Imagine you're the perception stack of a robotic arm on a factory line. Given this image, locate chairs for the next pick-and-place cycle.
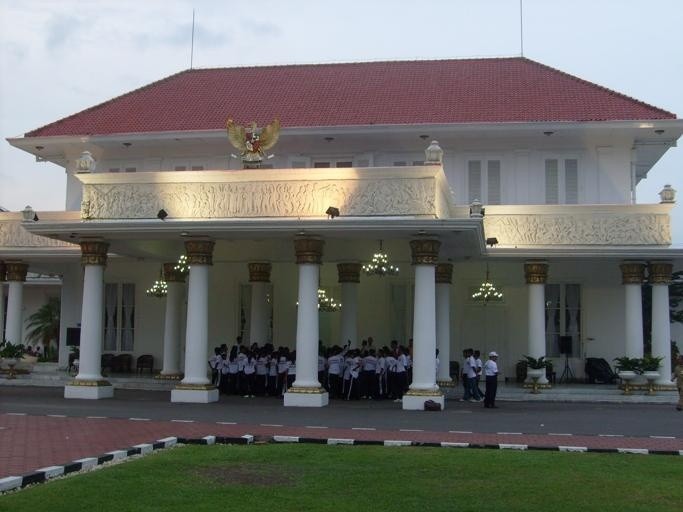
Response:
[67,352,153,375]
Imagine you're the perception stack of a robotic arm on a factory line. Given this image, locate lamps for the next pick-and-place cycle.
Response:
[469,259,504,307]
[156,209,168,221]
[142,261,168,299]
[484,237,498,248]
[325,206,339,220]
[294,264,343,313]
[359,239,400,278]
[173,244,189,277]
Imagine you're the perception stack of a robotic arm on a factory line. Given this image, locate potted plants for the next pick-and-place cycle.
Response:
[635,354,663,396]
[613,355,640,396]
[0,341,26,379]
[516,351,553,394]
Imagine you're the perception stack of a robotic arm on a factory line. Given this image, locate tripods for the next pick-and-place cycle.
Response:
[559,356,577,384]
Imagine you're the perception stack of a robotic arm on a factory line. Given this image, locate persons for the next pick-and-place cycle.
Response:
[25,345,34,354]
[670,355,683,410]
[208,336,413,404]
[33,346,43,361]
[459,348,485,403]
[483,351,499,408]
[436,349,439,384]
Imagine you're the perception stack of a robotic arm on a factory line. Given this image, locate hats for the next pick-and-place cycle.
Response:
[488,352,499,357]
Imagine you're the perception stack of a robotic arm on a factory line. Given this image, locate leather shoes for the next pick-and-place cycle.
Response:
[487,404,498,408]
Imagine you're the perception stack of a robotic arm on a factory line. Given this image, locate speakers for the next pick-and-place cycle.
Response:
[559,336,573,354]
[66,328,81,346]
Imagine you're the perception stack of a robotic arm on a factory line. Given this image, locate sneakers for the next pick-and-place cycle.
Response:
[329,392,403,404]
[460,394,485,402]
[217,389,283,400]
